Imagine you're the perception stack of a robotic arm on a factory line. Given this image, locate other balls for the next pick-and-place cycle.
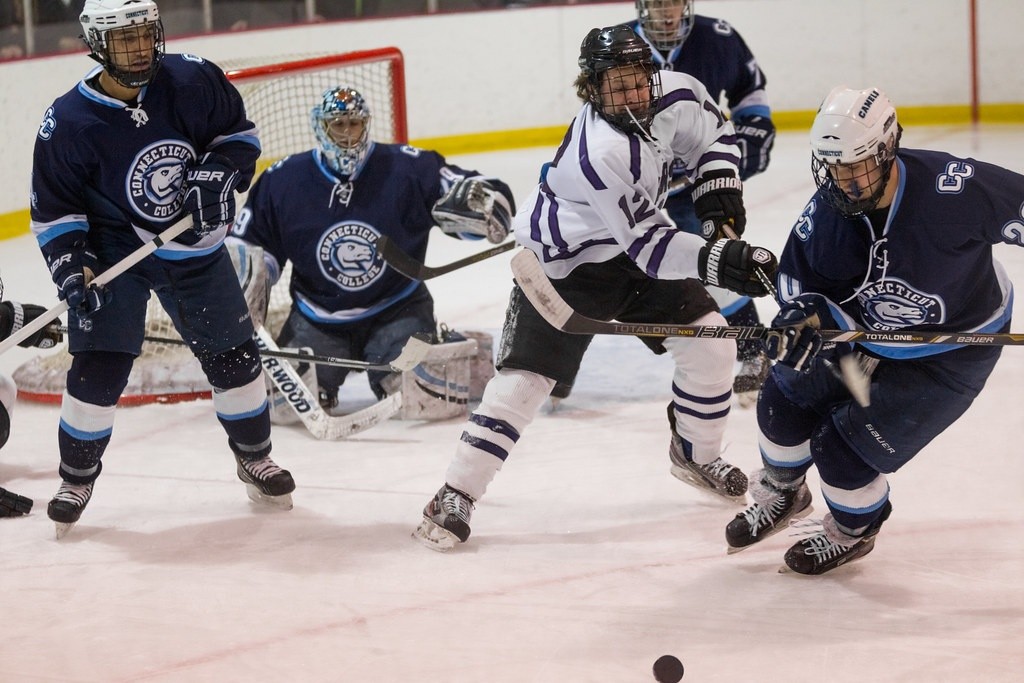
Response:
[652,654,685,683]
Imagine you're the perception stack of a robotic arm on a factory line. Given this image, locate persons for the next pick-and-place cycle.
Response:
[412,13,783,549]
[0,276,62,516]
[222,84,517,424]
[724,82,1023,576]
[26,0,296,539]
[620,0,776,408]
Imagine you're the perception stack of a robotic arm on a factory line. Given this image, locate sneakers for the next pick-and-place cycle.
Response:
[410,482,476,554]
[776,498,893,576]
[46,476,95,541]
[233,450,296,511]
[724,475,814,555]
[666,400,749,506]
[733,349,774,409]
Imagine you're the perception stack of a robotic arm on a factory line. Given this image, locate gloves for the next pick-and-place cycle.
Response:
[760,302,825,375]
[46,246,114,335]
[697,236,780,299]
[3,299,63,349]
[735,115,775,181]
[682,170,747,241]
[179,152,244,240]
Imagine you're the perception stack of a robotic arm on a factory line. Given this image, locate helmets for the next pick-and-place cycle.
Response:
[808,84,904,223]
[78,0,166,89]
[576,24,663,135]
[635,0,696,51]
[310,86,373,176]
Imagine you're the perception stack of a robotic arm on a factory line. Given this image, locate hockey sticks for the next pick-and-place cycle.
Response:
[374,180,691,285]
[46,321,437,375]
[250,320,404,445]
[1,211,196,358]
[510,244,1024,351]
[720,221,874,409]
